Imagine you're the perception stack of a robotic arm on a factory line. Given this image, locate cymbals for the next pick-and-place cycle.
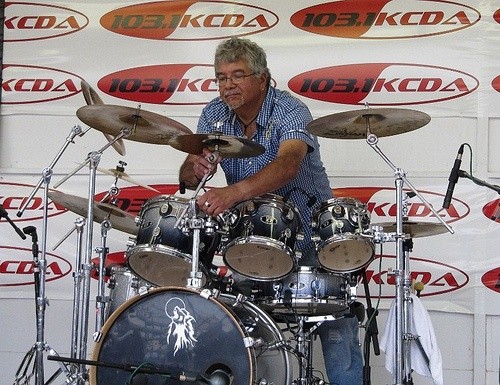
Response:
[80,80,127,156]
[75,161,162,193]
[167,133,267,158]
[48,193,141,235]
[305,109,431,139]
[368,222,452,238]
[76,105,193,145]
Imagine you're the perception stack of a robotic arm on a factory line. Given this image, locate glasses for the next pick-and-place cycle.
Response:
[213,72,255,86]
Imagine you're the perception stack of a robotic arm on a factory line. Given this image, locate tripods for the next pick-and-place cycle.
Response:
[13,125,90,385]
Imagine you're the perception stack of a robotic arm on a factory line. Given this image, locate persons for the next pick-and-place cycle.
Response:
[179,38,365,385]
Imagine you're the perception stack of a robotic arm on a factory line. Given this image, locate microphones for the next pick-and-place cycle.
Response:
[207,368,230,385]
[442,145,464,208]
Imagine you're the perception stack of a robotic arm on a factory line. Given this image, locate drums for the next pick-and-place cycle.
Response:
[221,191,302,282]
[91,285,294,385]
[103,267,151,324]
[310,196,375,273]
[123,194,222,289]
[251,265,354,323]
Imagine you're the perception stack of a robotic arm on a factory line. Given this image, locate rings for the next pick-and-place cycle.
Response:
[205,201,210,207]
[197,156,201,160]
